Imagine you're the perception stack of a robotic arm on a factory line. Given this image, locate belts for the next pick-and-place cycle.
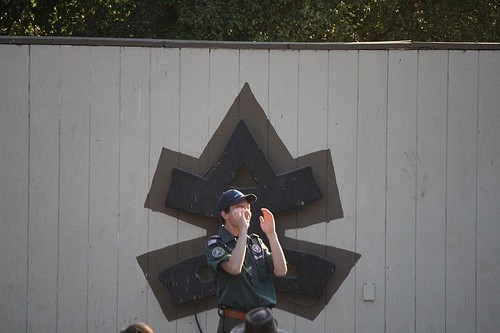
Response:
[224,309,246,320]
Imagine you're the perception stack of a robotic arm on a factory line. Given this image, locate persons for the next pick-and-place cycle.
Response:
[204,188,288,333]
[119,320,154,333]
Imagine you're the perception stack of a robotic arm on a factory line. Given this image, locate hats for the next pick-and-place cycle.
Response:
[230,307,291,333]
[218,189,257,211]
